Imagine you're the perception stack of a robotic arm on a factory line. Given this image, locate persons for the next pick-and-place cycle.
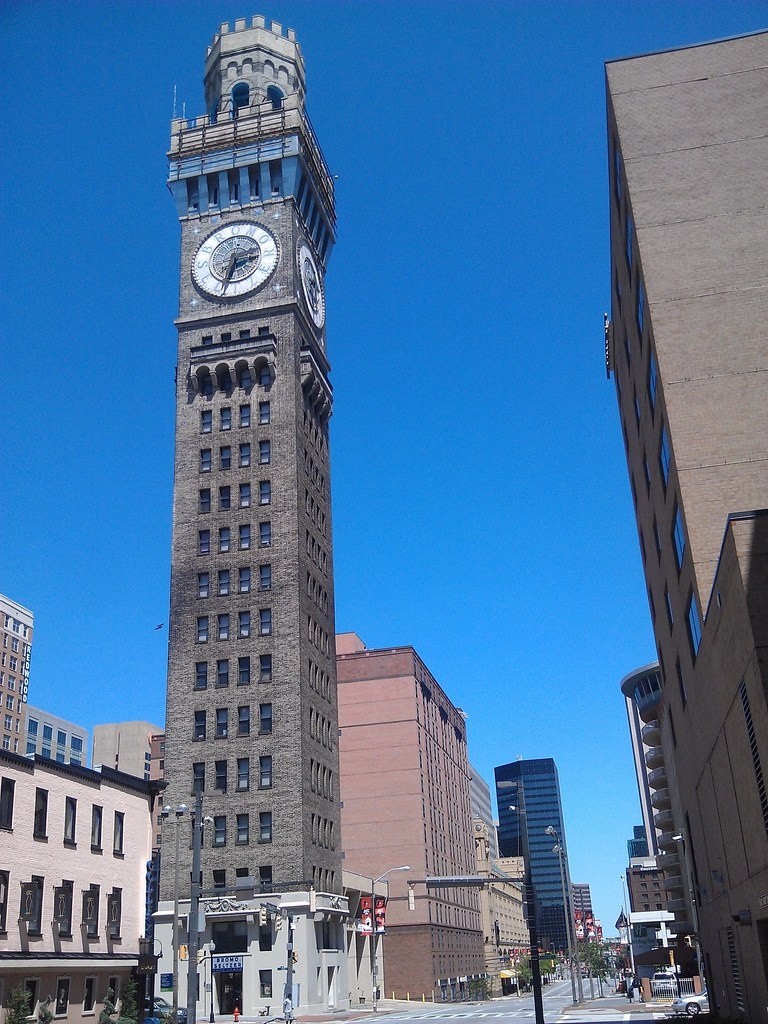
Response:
[283,993,294,1024]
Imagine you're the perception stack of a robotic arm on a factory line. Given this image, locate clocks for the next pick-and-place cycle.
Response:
[190,220,280,301]
[295,235,326,331]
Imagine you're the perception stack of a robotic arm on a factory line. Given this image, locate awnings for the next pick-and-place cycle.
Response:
[500,969,522,978]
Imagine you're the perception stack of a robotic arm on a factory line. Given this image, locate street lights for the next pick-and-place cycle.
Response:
[162,803,189,1023]
[208,939,217,1022]
[544,825,585,1007]
[371,866,410,1012]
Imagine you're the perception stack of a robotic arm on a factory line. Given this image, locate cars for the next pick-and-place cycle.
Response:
[143,994,187,1024]
[651,973,677,996]
[670,988,710,1015]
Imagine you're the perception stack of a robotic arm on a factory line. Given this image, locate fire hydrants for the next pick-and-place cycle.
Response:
[233,1006,241,1022]
